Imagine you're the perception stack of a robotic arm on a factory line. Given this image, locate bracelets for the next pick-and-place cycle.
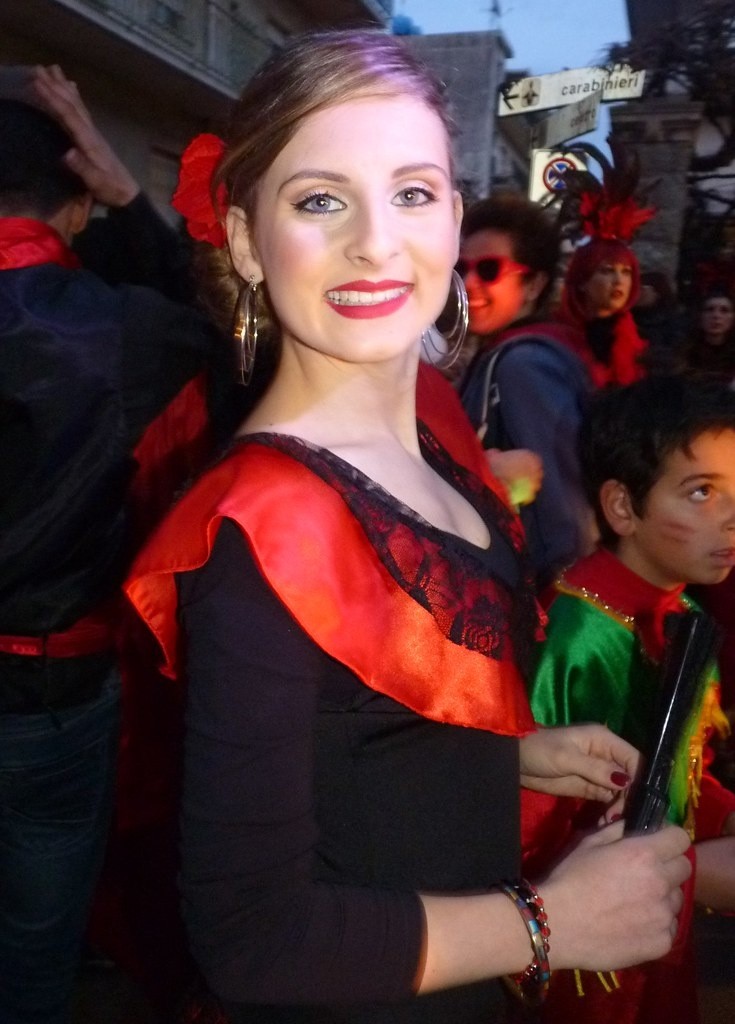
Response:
[489,882,551,1007]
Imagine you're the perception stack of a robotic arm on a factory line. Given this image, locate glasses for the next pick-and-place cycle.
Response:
[455,255,536,286]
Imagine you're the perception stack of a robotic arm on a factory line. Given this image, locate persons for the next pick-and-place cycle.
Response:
[530,372,735,1024]
[121,31,691,1024]
[1,65,224,1024]
[440,197,734,583]
